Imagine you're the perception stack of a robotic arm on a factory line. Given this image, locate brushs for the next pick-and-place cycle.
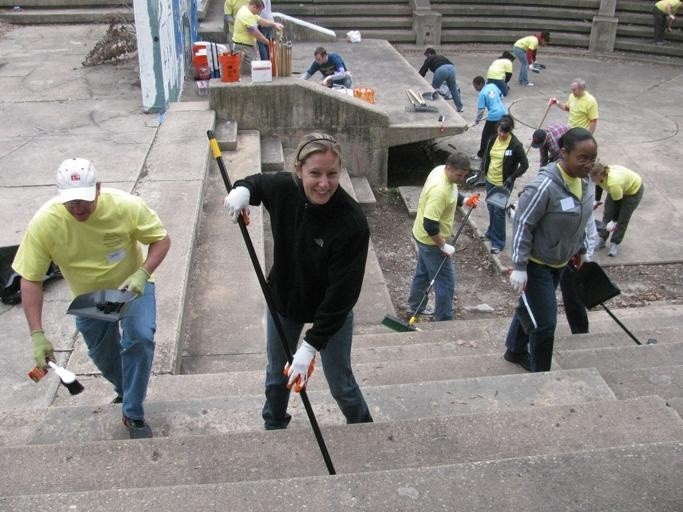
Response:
[45,357,84,395]
[516,291,538,332]
[438,113,446,122]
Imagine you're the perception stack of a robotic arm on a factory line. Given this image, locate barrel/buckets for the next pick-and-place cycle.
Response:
[195,49,208,78]
[251,60,272,82]
[192,42,208,65]
[219,54,240,82]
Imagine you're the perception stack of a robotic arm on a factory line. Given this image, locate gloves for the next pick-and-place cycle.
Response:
[118,267,151,297]
[551,97,560,106]
[222,184,251,225]
[594,199,602,210]
[533,60,538,65]
[439,241,457,258]
[459,192,481,209]
[605,220,617,232]
[29,329,58,371]
[503,176,514,186]
[282,340,317,392]
[508,270,531,294]
[572,252,591,269]
[529,64,535,70]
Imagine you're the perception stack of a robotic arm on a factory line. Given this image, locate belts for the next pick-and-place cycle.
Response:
[232,41,256,47]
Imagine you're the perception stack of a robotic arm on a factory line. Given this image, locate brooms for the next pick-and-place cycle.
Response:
[381,193,480,332]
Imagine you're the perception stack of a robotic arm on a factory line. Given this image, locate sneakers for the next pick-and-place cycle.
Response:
[459,104,465,112]
[406,302,437,316]
[595,237,607,252]
[607,241,618,257]
[479,232,490,242]
[503,347,531,372]
[121,413,153,440]
[470,152,483,161]
[525,82,535,86]
[110,396,123,404]
[491,244,502,254]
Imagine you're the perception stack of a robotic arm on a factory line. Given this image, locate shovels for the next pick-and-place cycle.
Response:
[574,262,644,346]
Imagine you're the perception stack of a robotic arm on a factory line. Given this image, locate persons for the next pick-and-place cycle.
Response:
[532,124,574,166]
[550,77,599,133]
[592,163,644,256]
[256,0,276,61]
[223,132,372,430]
[654,0,683,44]
[503,127,597,373]
[473,76,507,159]
[487,51,515,96]
[407,151,480,320]
[232,0,284,75]
[514,31,551,86]
[11,157,171,438]
[301,47,352,89]
[483,115,529,254]
[419,48,464,111]
[224,0,252,54]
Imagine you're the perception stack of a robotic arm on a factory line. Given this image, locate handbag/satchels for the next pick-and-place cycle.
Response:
[0,244,65,306]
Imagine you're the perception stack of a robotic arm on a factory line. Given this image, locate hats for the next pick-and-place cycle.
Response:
[54,157,101,204]
[530,128,549,149]
